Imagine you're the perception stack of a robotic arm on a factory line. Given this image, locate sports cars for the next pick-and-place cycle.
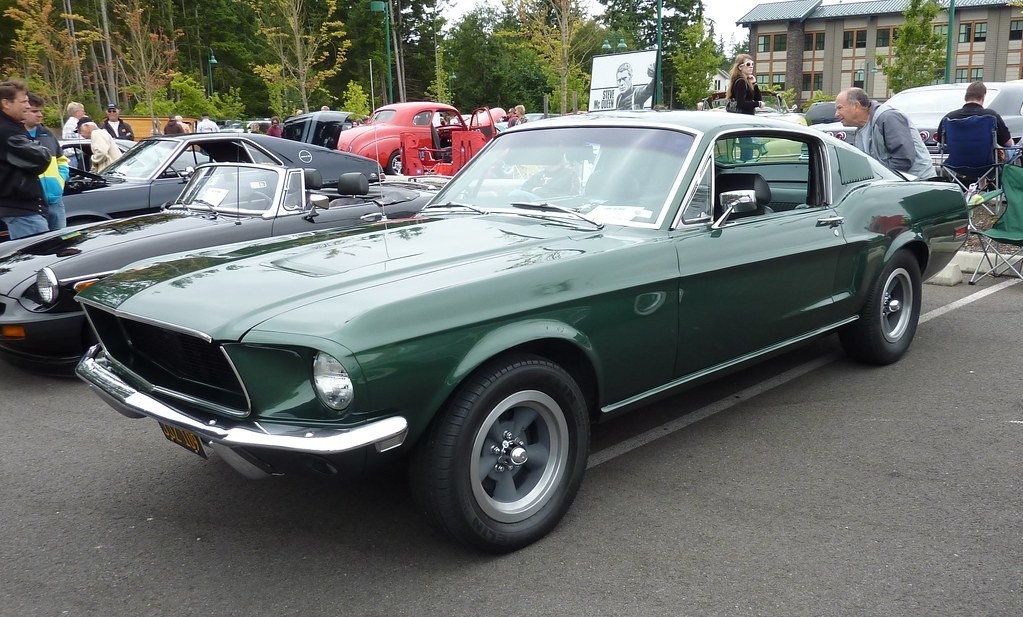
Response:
[0,163,454,372]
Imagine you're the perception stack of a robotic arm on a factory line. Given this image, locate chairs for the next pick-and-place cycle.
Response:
[937,113,1023,286]
[400,132,488,178]
[715,172,774,220]
[288,168,368,208]
[585,164,651,208]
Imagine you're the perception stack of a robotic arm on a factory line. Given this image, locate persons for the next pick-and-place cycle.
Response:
[937,81,1015,189]
[835,86,937,182]
[0,83,331,242]
[442,104,527,129]
[727,54,766,162]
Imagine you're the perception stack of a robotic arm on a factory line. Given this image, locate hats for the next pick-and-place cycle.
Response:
[202,113,210,116]
[77,118,92,129]
[107,103,120,109]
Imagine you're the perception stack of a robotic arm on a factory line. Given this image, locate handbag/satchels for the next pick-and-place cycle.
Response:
[726,78,747,112]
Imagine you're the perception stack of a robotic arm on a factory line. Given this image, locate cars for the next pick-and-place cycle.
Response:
[498,113,564,128]
[701,89,809,162]
[61,111,386,229]
[73,108,971,552]
[884,80,1023,204]
[56,138,218,175]
[804,99,882,147]
[336,106,508,176]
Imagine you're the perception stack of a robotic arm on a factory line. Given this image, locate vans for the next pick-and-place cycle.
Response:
[215,117,271,136]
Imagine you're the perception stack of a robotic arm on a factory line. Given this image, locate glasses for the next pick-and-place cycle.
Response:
[739,61,754,68]
[108,111,117,113]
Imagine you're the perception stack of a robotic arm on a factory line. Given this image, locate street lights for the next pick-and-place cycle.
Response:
[207,48,219,97]
[371,1,393,104]
[601,30,627,54]
[449,67,457,103]
[858,61,878,93]
